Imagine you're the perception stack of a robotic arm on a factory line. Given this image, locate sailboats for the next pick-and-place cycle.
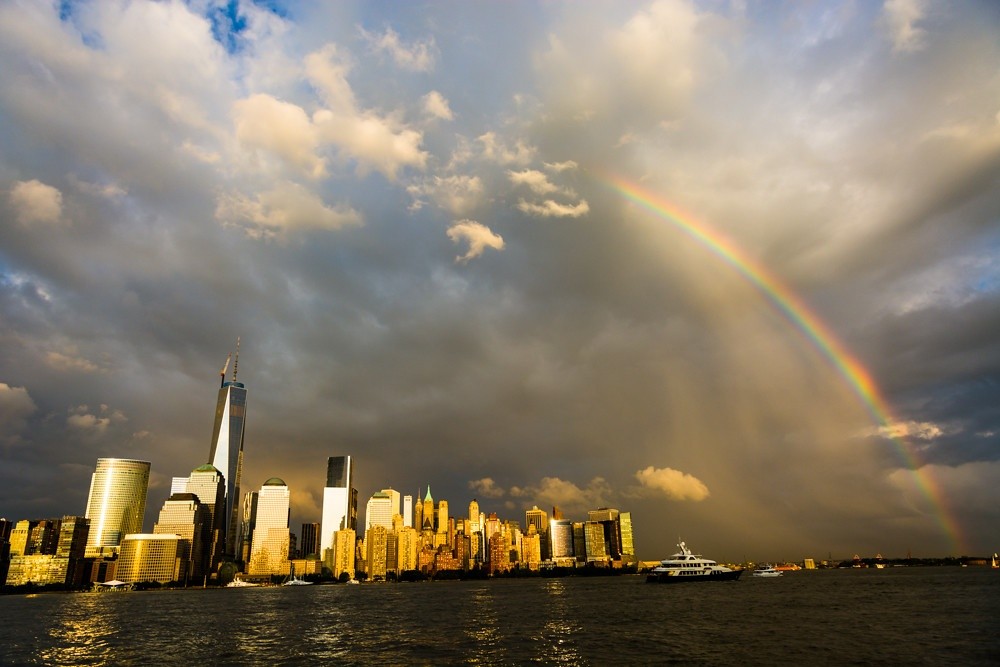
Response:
[991,556,998,569]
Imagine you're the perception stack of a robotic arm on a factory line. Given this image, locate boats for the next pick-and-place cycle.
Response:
[647,537,743,582]
[752,563,783,577]
[962,565,967,568]
